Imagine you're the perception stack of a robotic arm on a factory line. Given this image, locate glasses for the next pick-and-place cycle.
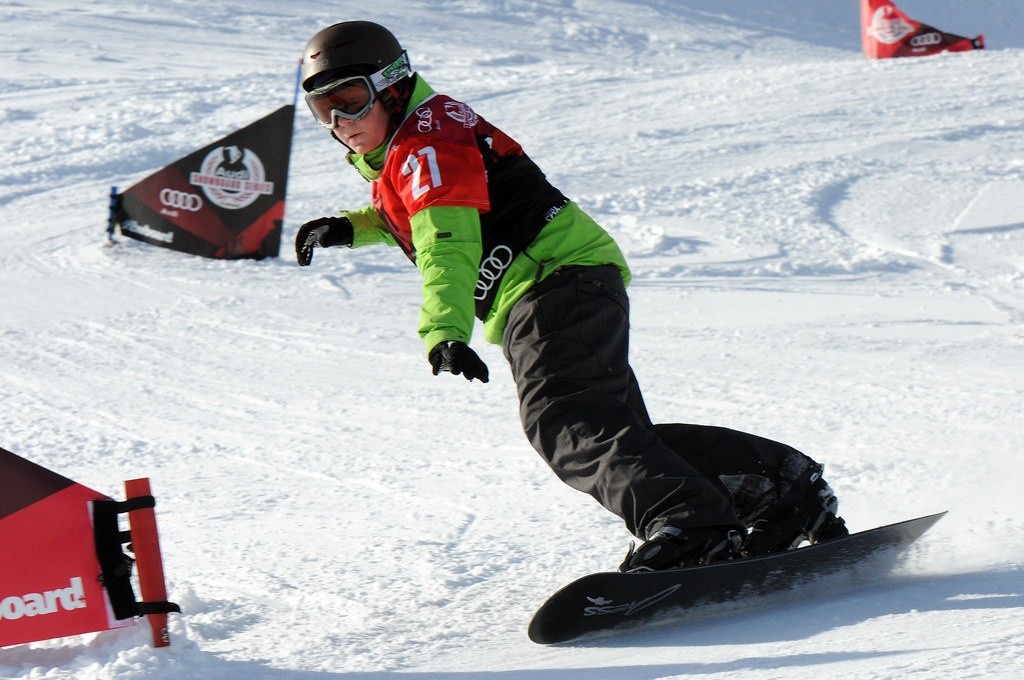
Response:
[305,76,375,129]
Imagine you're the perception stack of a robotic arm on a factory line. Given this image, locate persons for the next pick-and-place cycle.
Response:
[297,19,849,574]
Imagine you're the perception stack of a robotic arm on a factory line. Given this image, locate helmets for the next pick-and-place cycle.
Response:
[301,20,412,121]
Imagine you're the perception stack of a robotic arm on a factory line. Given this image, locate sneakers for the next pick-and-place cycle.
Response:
[741,474,848,558]
[619,526,743,573]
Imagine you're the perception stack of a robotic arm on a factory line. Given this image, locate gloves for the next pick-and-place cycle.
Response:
[430,340,489,383]
[296,216,353,266]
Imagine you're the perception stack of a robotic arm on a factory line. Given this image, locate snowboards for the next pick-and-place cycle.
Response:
[526,508,950,645]
